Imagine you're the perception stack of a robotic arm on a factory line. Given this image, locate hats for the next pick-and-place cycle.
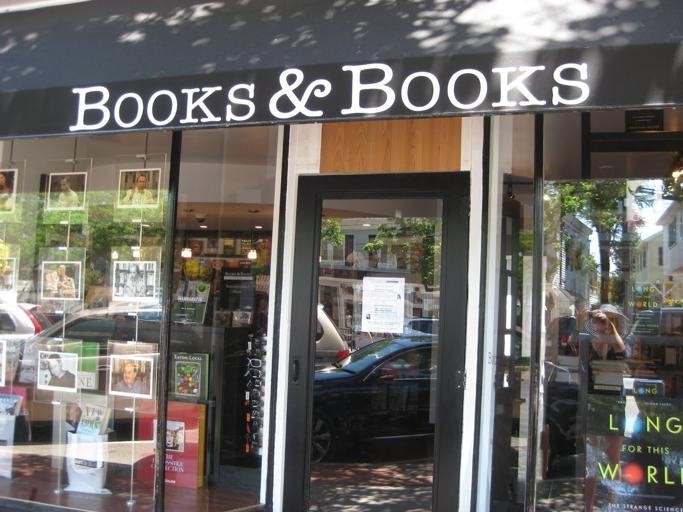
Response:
[583,304,631,341]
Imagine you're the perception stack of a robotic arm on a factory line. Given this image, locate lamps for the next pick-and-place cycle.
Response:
[180,232,192,257]
[246,233,260,260]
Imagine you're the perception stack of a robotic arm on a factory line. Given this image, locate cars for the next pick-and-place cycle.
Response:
[0,303,163,423]
[310,306,440,464]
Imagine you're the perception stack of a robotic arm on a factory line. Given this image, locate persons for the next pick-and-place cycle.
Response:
[0,173,12,193]
[165,430,180,451]
[124,175,153,204]
[567,304,632,512]
[124,264,146,296]
[116,363,144,394]
[48,355,75,387]
[59,177,78,208]
[52,265,75,297]
